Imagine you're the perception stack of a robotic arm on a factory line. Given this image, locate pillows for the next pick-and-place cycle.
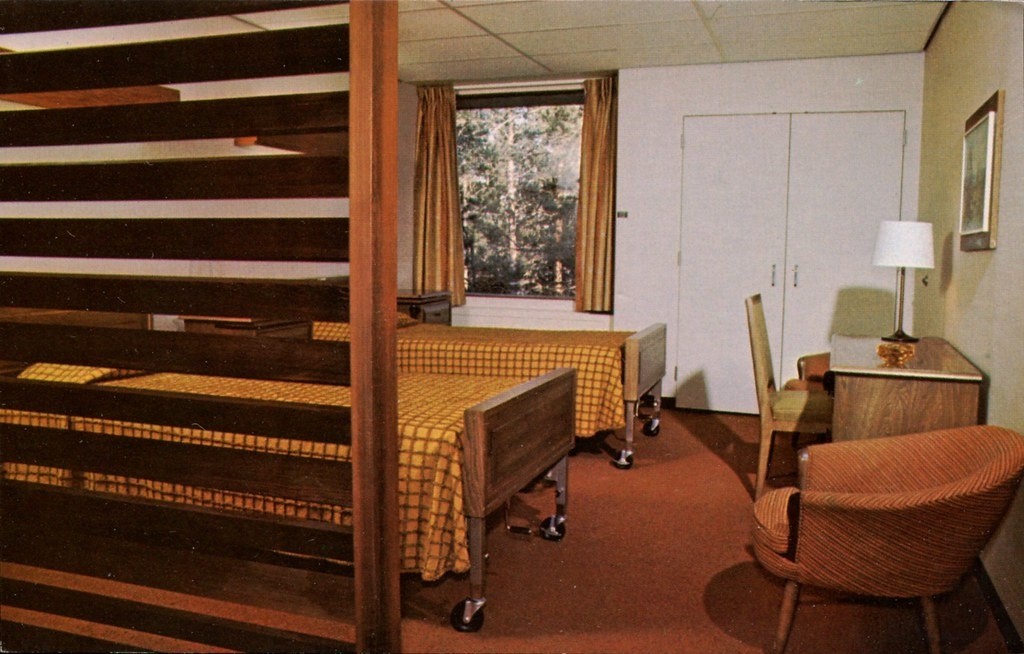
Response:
[18,362,153,386]
[313,311,416,339]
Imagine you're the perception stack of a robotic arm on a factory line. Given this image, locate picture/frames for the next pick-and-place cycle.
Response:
[959,89,1005,251]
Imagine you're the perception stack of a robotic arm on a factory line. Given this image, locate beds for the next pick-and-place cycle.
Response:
[313,311,667,468]
[0,306,577,631]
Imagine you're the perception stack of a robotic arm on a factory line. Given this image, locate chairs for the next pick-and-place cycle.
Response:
[745,293,834,500]
[750,425,1024,654]
[784,351,832,449]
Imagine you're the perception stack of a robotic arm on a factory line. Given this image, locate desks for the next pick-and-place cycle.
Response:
[830,335,983,442]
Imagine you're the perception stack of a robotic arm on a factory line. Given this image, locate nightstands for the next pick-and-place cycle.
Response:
[180,315,313,340]
[396,289,451,325]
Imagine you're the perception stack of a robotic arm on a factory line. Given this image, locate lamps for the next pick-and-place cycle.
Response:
[872,221,934,342]
